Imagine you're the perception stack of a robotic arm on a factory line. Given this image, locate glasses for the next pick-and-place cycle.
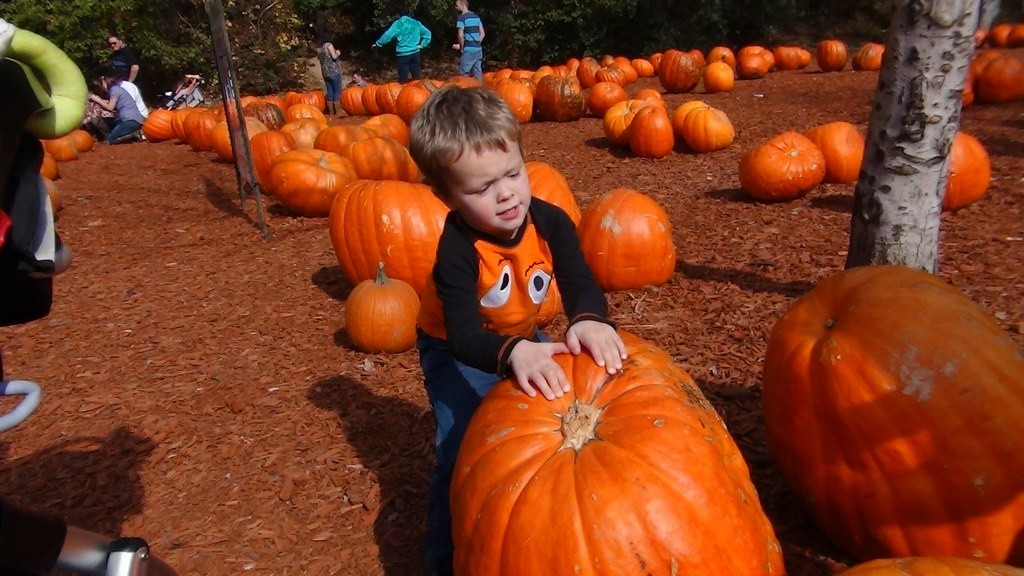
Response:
[108,42,120,46]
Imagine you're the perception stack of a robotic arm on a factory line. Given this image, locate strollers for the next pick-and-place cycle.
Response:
[157,73,206,109]
[0,22,153,575]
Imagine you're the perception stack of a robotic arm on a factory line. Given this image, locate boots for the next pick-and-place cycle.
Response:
[327,100,349,118]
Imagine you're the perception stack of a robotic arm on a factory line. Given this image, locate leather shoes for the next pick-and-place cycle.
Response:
[132,130,143,142]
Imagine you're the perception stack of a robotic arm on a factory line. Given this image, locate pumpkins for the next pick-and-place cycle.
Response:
[449,331,787,576]
[39,23,1024,351]
[762,264,1024,576]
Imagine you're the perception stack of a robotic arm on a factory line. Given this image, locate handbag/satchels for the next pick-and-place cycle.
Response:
[482,45,487,61]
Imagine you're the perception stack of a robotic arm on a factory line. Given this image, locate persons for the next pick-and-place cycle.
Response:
[372,5,431,83]
[89,72,149,145]
[408,85,628,576]
[318,42,341,116]
[107,33,142,98]
[452,0,485,80]
[83,94,109,143]
[346,70,369,87]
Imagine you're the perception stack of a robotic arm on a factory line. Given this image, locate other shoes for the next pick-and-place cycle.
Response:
[422,542,454,576]
